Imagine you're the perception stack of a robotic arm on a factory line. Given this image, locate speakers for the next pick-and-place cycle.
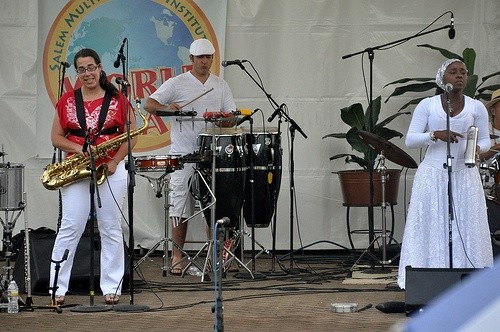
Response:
[404,265,484,317]
[14,231,132,292]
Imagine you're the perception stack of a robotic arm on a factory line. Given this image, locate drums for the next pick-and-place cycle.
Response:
[241,126,282,229]
[0,162,25,211]
[197,127,248,229]
[133,155,184,171]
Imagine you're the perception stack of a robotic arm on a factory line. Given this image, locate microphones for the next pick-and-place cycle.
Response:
[235,109,258,125]
[216,217,231,226]
[116,76,131,87]
[61,62,70,68]
[114,37,126,67]
[222,59,248,67]
[449,13,455,39]
[267,104,285,123]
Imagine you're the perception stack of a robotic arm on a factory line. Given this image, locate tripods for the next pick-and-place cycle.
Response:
[130,168,209,281]
[342,23,451,275]
[180,120,253,282]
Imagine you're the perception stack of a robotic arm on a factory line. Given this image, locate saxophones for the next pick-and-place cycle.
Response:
[40,96,149,190]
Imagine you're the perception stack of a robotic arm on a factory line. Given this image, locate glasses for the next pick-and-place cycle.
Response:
[77,64,100,74]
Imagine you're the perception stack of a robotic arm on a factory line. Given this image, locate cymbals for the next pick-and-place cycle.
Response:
[355,129,418,169]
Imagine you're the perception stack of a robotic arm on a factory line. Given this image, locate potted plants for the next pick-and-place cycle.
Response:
[321,96,418,207]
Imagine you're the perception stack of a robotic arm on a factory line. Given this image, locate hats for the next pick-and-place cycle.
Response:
[190,39,215,56]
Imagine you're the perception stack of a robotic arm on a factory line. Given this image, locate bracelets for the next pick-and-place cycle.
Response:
[431,131,437,141]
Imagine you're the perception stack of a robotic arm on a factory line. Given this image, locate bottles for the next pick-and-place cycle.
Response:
[7,281,19,313]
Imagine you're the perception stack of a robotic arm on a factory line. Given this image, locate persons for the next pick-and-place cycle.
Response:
[479,88,500,233]
[49,48,138,305]
[397,59,494,289]
[144,38,238,276]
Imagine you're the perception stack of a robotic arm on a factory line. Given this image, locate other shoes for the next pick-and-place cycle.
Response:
[105,294,120,304]
[51,294,64,305]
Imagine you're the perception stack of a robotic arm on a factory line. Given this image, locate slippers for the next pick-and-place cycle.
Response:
[229,262,240,272]
[170,268,182,275]
